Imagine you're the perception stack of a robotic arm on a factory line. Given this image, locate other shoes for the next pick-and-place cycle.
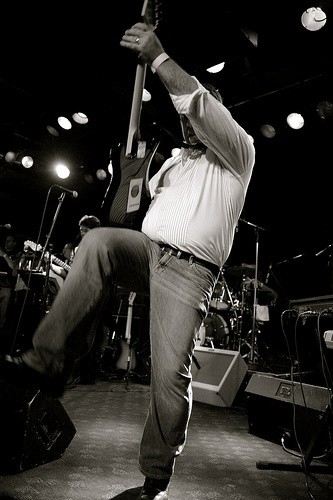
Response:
[0,353,68,398]
[140,476,171,500]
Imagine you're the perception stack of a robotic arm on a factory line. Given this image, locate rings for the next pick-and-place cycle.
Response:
[136,37,139,43]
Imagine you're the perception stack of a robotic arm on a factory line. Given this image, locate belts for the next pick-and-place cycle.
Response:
[160,245,219,278]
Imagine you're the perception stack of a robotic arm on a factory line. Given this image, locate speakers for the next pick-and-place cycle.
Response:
[184,345,248,409]
[245,373,333,465]
[0,377,75,475]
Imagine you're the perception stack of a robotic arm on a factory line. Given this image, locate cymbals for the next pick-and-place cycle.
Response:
[226,266,259,278]
[244,289,272,293]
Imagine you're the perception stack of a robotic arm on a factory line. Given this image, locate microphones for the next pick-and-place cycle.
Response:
[54,183,78,198]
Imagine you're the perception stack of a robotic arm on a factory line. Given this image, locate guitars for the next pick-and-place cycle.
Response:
[23,240,70,271]
[108,0,162,226]
[116,291,136,370]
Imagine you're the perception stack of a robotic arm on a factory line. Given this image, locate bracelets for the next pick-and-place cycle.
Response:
[151,52,170,73]
[3,254,7,257]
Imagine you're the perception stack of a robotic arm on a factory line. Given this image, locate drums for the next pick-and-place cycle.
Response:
[195,313,229,348]
[210,281,233,310]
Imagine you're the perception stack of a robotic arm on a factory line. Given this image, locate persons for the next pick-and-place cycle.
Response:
[1,23,255,499]
[1,215,101,271]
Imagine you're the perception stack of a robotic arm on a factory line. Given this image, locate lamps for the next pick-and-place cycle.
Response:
[4,2,329,180]
